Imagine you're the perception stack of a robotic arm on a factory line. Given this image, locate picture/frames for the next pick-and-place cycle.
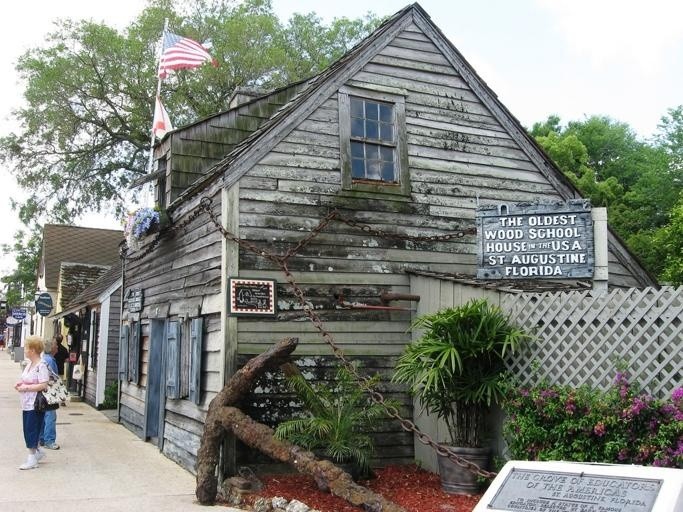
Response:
[224,274,278,321]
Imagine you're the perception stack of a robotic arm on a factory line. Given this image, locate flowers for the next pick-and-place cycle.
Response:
[117,200,161,255]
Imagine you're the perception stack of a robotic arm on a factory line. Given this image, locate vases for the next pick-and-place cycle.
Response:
[143,220,160,236]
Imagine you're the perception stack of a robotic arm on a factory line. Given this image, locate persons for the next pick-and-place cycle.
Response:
[15,334,50,471]
[54,334,70,376]
[0,331,5,351]
[40,338,60,449]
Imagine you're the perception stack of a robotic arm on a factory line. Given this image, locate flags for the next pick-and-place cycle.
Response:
[153,96,174,140]
[155,31,220,79]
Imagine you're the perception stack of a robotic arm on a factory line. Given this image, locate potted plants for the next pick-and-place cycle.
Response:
[384,294,547,497]
[269,357,403,493]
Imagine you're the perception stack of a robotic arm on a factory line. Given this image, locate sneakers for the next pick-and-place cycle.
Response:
[19,447,44,471]
[43,440,60,450]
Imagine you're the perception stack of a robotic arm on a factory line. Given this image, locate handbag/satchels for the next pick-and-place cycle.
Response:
[34,388,60,412]
[37,361,71,405]
